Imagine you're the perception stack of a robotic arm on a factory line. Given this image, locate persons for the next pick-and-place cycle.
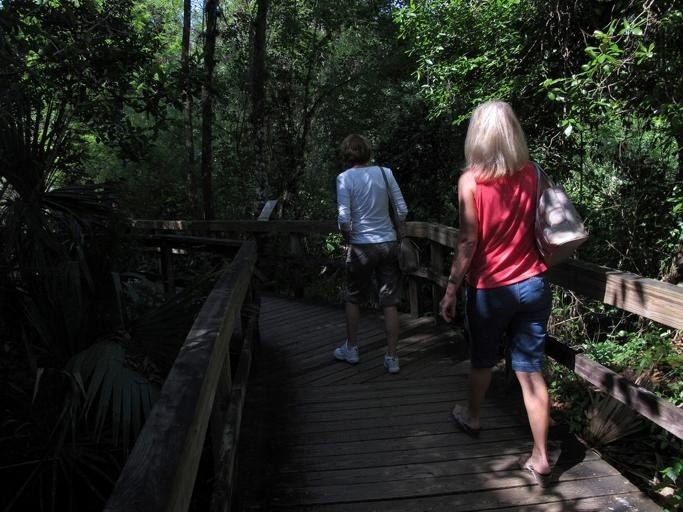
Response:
[334,134,408,374]
[438,99,552,479]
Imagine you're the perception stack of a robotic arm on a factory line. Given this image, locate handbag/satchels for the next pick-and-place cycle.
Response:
[396,238,423,273]
[534,186,590,267]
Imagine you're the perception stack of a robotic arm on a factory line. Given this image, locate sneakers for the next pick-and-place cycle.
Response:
[333,338,360,364]
[384,355,400,373]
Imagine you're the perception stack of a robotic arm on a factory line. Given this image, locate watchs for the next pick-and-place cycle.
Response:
[448,274,459,285]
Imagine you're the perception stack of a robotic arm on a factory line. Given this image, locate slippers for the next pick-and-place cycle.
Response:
[517,454,553,488]
[451,403,480,438]
